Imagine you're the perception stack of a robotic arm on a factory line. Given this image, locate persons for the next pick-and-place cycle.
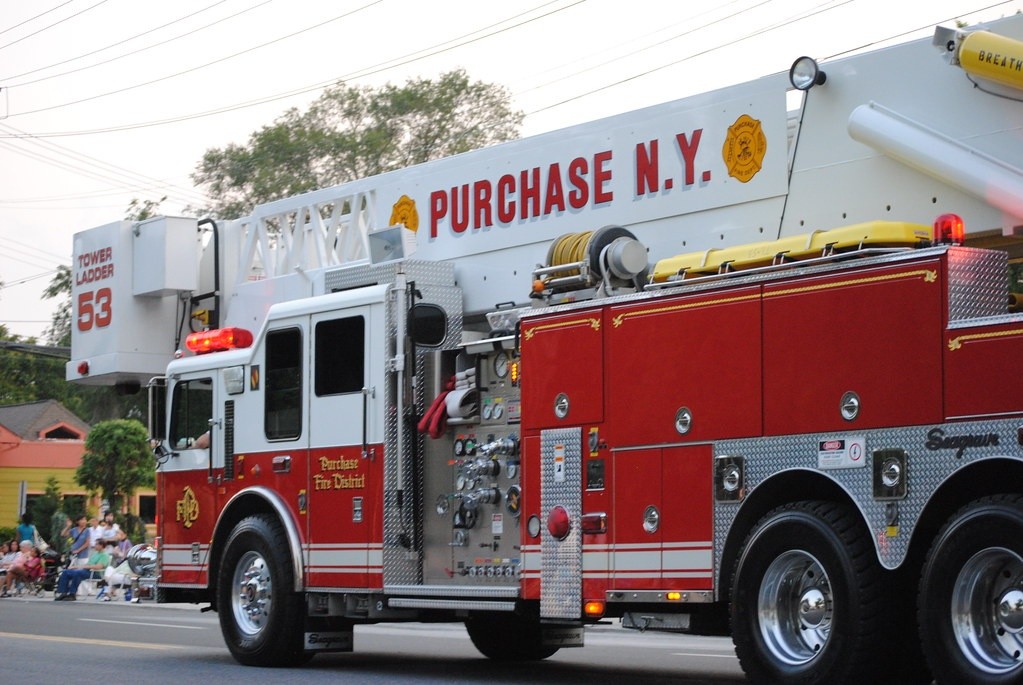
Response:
[0,511,135,602]
[188,430,213,451]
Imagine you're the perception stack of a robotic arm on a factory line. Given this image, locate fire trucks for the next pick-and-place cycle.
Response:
[64,7,1023,685]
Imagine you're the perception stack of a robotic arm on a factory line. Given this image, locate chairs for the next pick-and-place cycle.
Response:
[97,559,134,602]
[75,554,112,601]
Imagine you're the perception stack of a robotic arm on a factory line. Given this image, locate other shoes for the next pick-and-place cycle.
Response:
[55,593,67,601]
[1,591,12,597]
[63,594,76,601]
[100,596,111,601]
